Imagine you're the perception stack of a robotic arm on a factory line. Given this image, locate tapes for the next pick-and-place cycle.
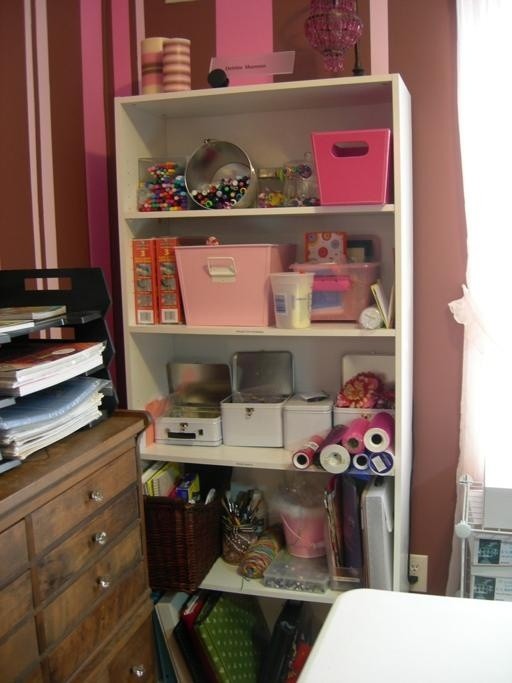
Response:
[290,412,395,476]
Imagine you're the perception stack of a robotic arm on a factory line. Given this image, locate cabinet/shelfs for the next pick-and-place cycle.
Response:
[0,267,119,479]
[0,410,155,683]
[114,71,412,683]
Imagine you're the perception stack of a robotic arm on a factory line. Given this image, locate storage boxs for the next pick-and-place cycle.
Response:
[154,359,232,447]
[172,244,297,326]
[310,128,391,206]
[333,351,395,427]
[287,261,382,323]
[283,391,336,450]
[220,350,294,447]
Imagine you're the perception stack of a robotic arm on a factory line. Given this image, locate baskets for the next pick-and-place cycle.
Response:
[143,495,222,596]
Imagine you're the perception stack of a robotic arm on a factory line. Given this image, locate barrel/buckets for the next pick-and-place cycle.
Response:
[183,137,260,209]
[277,495,329,560]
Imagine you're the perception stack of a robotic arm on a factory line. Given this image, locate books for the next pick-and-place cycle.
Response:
[132,237,159,325]
[150,588,317,683]
[0,306,67,321]
[0,376,104,460]
[0,320,35,333]
[0,339,106,396]
[297,231,373,316]
[370,278,395,329]
[155,234,208,324]
[140,461,184,497]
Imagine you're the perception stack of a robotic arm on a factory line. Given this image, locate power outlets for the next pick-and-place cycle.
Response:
[408,553,428,594]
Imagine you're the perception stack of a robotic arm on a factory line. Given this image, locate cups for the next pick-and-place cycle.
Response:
[271,274,312,329]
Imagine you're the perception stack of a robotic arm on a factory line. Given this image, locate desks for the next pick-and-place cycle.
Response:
[295,588,512,683]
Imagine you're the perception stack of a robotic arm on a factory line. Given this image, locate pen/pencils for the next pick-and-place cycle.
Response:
[138,161,188,212]
[221,486,263,516]
[190,175,251,209]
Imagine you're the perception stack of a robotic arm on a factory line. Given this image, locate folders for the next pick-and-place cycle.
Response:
[178,589,206,638]
